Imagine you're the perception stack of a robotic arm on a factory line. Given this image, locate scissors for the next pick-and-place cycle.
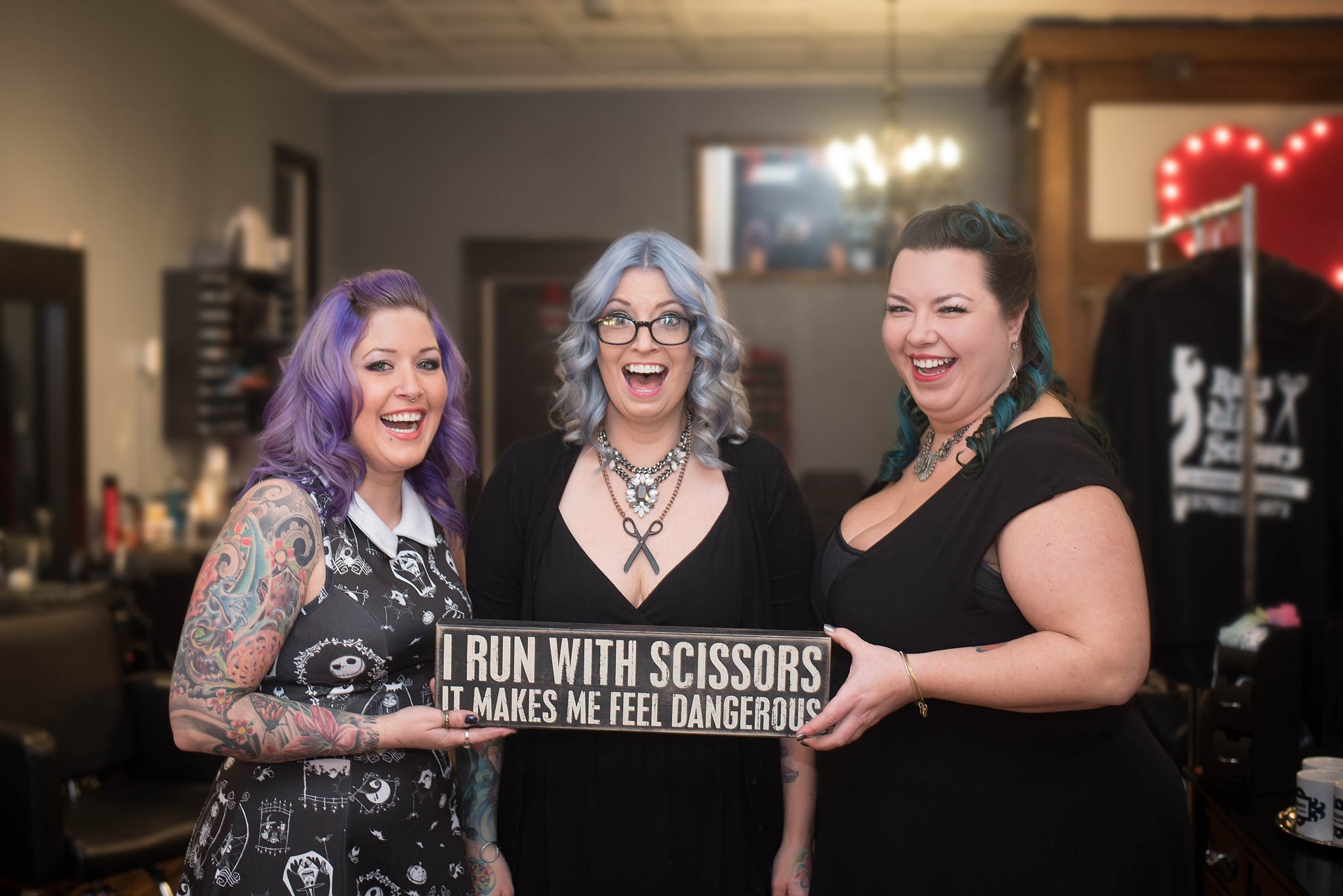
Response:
[623,517,663,575]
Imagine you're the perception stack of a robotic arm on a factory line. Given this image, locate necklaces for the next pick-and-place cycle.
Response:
[597,410,693,574]
[912,420,975,481]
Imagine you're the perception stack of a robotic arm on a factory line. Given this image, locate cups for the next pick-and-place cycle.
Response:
[1296,756,1343,845]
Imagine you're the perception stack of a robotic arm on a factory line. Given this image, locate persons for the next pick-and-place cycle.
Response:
[457,231,818,896]
[167,268,517,896]
[795,199,1185,896]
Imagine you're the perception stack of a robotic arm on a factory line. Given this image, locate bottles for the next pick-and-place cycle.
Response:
[100,474,186,557]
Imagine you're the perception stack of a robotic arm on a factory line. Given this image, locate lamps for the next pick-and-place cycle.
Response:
[822,0,967,213]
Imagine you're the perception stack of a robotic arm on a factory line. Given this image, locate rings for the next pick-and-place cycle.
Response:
[444,709,450,729]
[463,728,470,748]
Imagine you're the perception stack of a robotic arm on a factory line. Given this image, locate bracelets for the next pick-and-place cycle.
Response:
[898,651,922,715]
[479,841,502,863]
[903,653,928,717]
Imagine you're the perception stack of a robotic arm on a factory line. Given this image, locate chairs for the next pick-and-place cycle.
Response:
[0,610,218,896]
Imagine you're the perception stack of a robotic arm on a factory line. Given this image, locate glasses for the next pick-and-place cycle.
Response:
[590,314,698,346]
